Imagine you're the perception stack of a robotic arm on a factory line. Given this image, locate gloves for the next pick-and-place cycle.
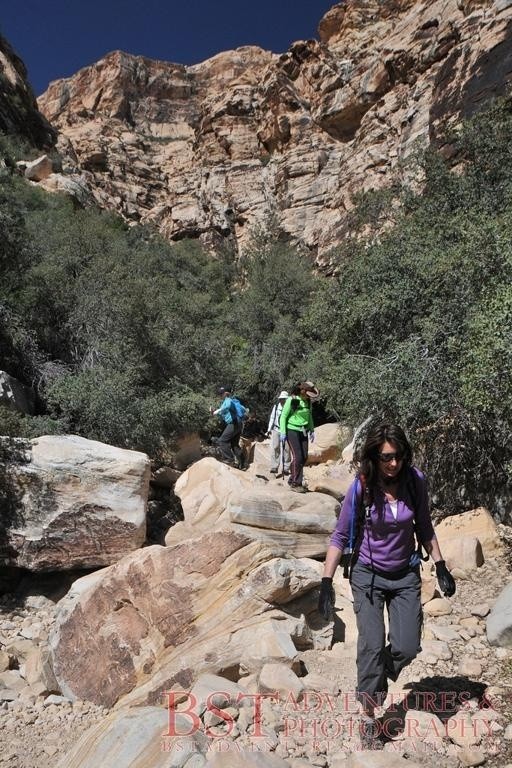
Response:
[280,434,287,441]
[435,560,456,597]
[310,432,315,443]
[318,577,336,621]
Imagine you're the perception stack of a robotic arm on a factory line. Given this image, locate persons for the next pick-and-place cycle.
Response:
[280,381,319,493]
[265,391,291,476]
[234,410,268,470]
[213,392,245,470]
[320,424,456,744]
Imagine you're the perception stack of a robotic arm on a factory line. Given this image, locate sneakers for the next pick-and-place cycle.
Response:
[288,480,308,493]
[359,713,384,751]
[222,459,234,466]
[233,463,240,468]
[284,469,290,475]
[270,468,278,473]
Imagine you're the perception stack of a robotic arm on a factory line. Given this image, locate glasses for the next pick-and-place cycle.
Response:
[378,452,402,462]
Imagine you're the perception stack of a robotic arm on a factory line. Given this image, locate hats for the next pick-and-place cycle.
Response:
[278,390,288,399]
[217,385,230,395]
